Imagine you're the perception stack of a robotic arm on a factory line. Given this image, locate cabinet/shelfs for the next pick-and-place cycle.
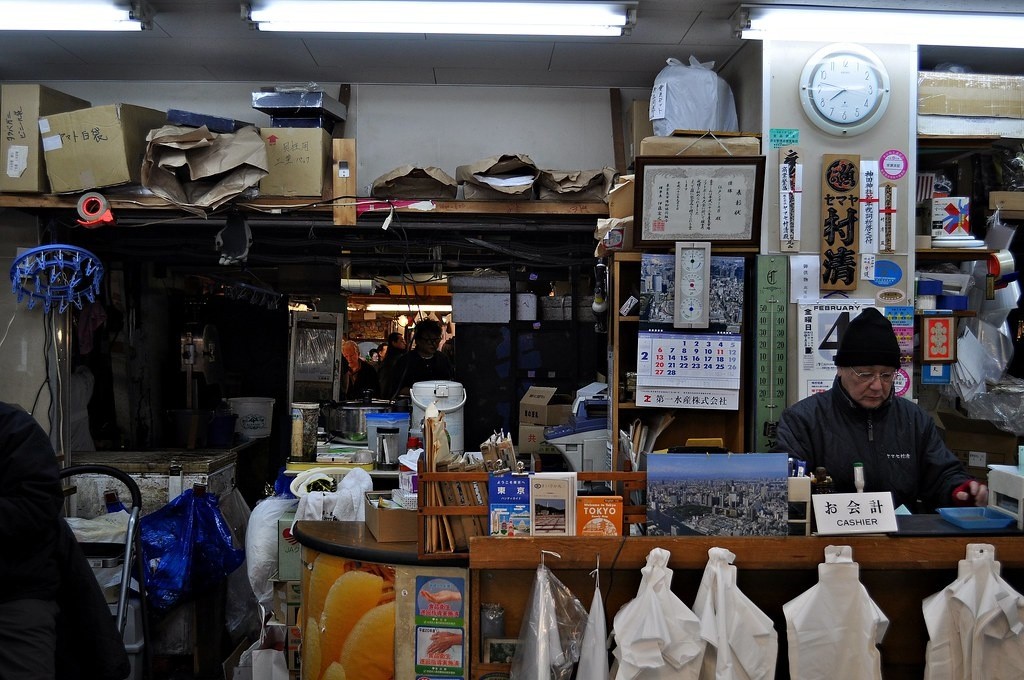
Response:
[606,252,748,496]
[915,248,1001,319]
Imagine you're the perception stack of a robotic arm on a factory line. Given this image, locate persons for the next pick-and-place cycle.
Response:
[426,631,462,655]
[421,589,462,603]
[340,319,454,399]
[0,401,65,680]
[774,307,989,515]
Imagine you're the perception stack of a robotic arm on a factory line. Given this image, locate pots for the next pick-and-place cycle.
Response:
[320,389,395,440]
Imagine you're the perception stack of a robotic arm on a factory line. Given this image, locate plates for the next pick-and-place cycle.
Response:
[936,507,1014,529]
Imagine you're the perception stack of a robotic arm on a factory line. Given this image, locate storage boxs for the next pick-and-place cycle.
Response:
[266,614,301,671]
[519,372,605,425]
[222,637,252,680]
[272,581,301,625]
[916,196,970,236]
[915,234,932,249]
[258,128,332,197]
[39,103,166,195]
[278,506,301,580]
[364,491,419,542]
[572,296,597,321]
[451,292,537,322]
[446,260,527,294]
[624,97,654,169]
[0,84,91,194]
[519,421,563,454]
[69,450,238,519]
[538,296,572,320]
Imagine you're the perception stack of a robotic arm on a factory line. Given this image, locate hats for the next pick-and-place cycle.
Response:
[834,307,901,369]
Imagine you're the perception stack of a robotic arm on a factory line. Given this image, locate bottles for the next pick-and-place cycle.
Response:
[593,290,608,333]
[290,402,320,460]
[408,429,423,460]
[596,264,605,285]
[376,427,400,471]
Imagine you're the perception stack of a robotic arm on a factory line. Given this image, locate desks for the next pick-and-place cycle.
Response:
[283,468,418,480]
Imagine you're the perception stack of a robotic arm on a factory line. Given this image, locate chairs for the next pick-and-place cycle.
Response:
[623,460,646,537]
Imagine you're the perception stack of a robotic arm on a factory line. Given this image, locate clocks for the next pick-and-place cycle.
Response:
[797,41,891,138]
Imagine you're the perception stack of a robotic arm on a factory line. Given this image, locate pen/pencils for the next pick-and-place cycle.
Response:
[788,458,793,477]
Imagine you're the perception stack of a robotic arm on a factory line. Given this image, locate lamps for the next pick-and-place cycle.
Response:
[341,279,376,297]
[728,3,1024,49]
[591,287,607,313]
[240,4,636,38]
[0,0,156,31]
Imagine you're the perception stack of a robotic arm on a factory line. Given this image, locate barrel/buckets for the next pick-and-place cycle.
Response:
[222,397,276,439]
[365,413,410,456]
[410,380,467,455]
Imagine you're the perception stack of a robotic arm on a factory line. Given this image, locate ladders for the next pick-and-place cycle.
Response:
[57,465,158,680]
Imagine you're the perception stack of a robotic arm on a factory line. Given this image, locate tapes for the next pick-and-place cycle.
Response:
[77,192,111,221]
[987,249,1015,281]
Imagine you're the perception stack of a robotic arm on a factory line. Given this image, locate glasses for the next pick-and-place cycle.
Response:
[422,337,440,345]
[849,366,898,382]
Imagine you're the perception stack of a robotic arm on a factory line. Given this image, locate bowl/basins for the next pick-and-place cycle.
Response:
[162,410,239,448]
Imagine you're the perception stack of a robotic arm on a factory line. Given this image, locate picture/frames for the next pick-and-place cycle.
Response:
[632,154,766,248]
